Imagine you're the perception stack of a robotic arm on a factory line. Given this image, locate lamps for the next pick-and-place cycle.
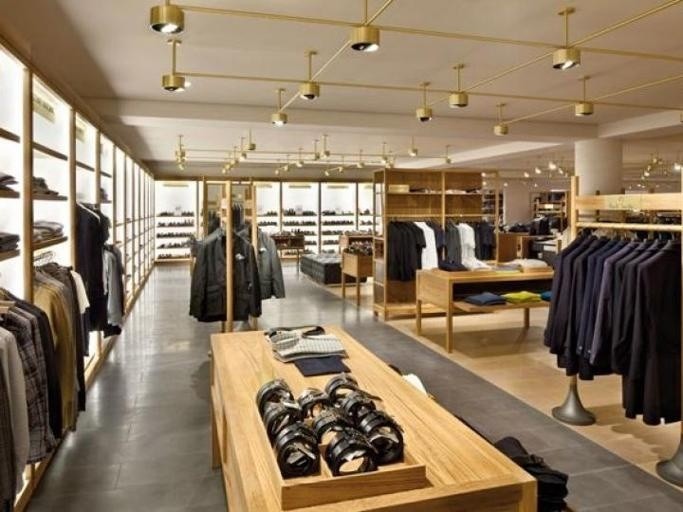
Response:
[270,89,287,127]
[162,38,185,92]
[150,0,185,34]
[416,81,433,123]
[494,104,509,137]
[300,52,321,102]
[449,63,469,108]
[174,128,453,176]
[575,76,594,116]
[554,8,580,68]
[351,0,379,52]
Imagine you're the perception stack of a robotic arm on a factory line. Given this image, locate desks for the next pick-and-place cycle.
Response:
[209,324,540,512]
[416,266,557,354]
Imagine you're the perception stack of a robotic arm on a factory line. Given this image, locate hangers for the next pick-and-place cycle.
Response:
[582,218,676,243]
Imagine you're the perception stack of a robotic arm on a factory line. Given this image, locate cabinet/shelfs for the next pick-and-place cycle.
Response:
[1,44,154,314]
[154,170,569,263]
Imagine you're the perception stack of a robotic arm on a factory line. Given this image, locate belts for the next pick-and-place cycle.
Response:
[257,373,403,476]
